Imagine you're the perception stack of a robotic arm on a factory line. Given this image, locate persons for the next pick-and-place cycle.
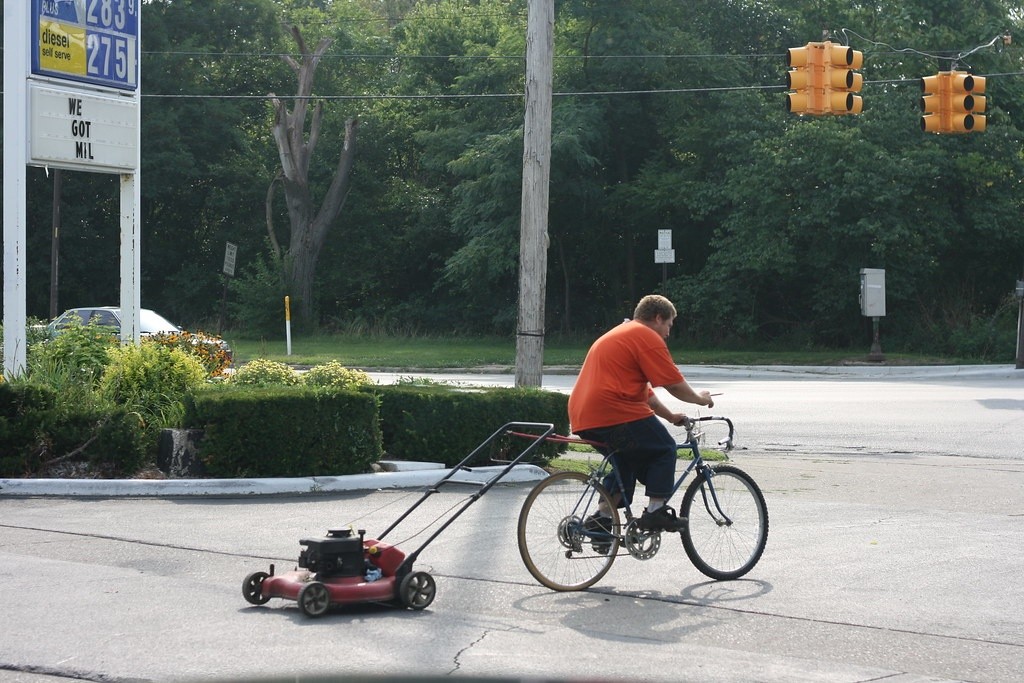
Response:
[568,294,714,554]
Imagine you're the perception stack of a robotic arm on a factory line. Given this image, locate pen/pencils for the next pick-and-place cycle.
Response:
[710,393,724,396]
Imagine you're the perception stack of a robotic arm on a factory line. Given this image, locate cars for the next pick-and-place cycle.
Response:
[29,307,234,364]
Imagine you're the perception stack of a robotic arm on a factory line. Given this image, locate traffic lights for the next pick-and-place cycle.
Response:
[785,45,814,113]
[949,69,974,134]
[823,41,853,116]
[845,49,863,115]
[919,71,947,135]
[968,74,987,133]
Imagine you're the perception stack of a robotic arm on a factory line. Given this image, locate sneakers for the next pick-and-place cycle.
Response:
[639,505,688,529]
[585,510,612,547]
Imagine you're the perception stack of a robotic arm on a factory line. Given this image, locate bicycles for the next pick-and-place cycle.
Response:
[517,415,770,592]
